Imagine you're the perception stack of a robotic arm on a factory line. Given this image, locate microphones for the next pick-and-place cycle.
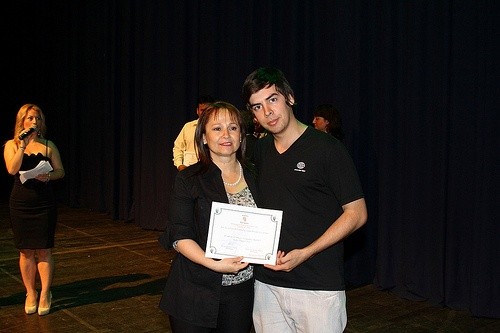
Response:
[19,127,39,140]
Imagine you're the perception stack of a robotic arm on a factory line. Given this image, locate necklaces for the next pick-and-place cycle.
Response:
[221,159,243,187]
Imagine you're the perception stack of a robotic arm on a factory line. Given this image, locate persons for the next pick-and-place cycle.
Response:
[240,64,368,332]
[3,103,66,316]
[160,103,263,333]
[310,104,342,140]
[170,93,217,172]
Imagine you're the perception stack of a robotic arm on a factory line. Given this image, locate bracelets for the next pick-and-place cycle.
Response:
[47,172,51,182]
[19,146,26,151]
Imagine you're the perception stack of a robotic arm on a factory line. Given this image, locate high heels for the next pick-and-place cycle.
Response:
[37,289,53,314]
[25,292,38,313]
